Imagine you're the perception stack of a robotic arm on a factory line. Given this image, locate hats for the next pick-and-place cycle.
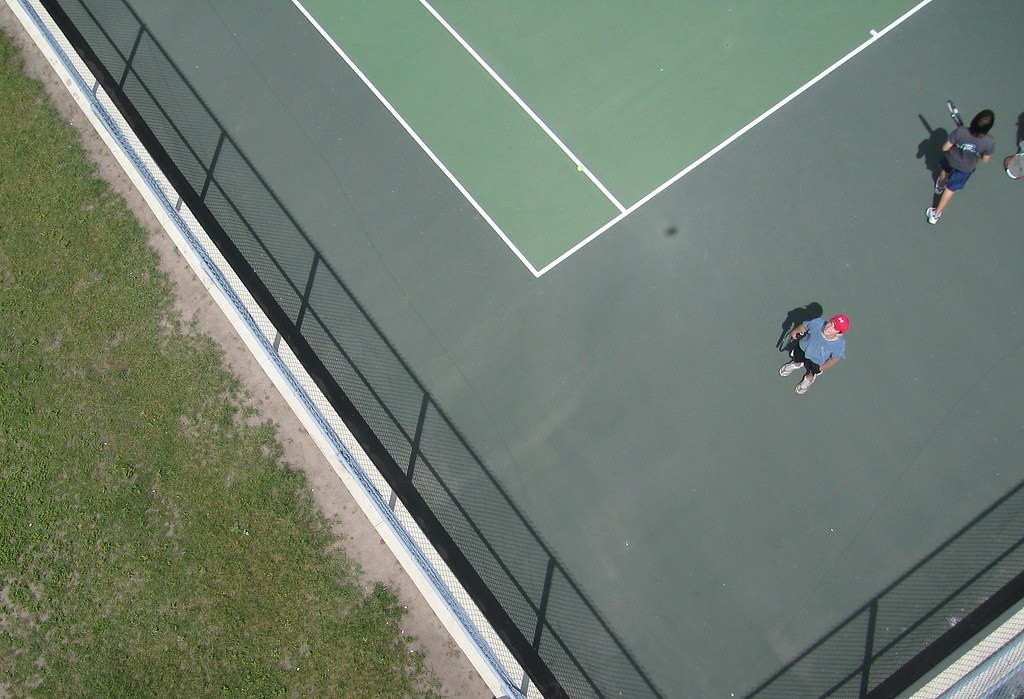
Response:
[831,315,850,334]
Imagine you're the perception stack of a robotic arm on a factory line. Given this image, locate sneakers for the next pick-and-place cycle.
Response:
[935,176,948,195]
[795,375,816,394]
[926,207,941,224]
[779,361,804,376]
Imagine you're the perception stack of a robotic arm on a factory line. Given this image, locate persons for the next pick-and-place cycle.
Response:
[778,314,850,394]
[926,109,996,225]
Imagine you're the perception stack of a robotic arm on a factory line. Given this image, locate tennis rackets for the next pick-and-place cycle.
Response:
[946,100,964,127]
[779,332,804,352]
[1006,152,1024,180]
